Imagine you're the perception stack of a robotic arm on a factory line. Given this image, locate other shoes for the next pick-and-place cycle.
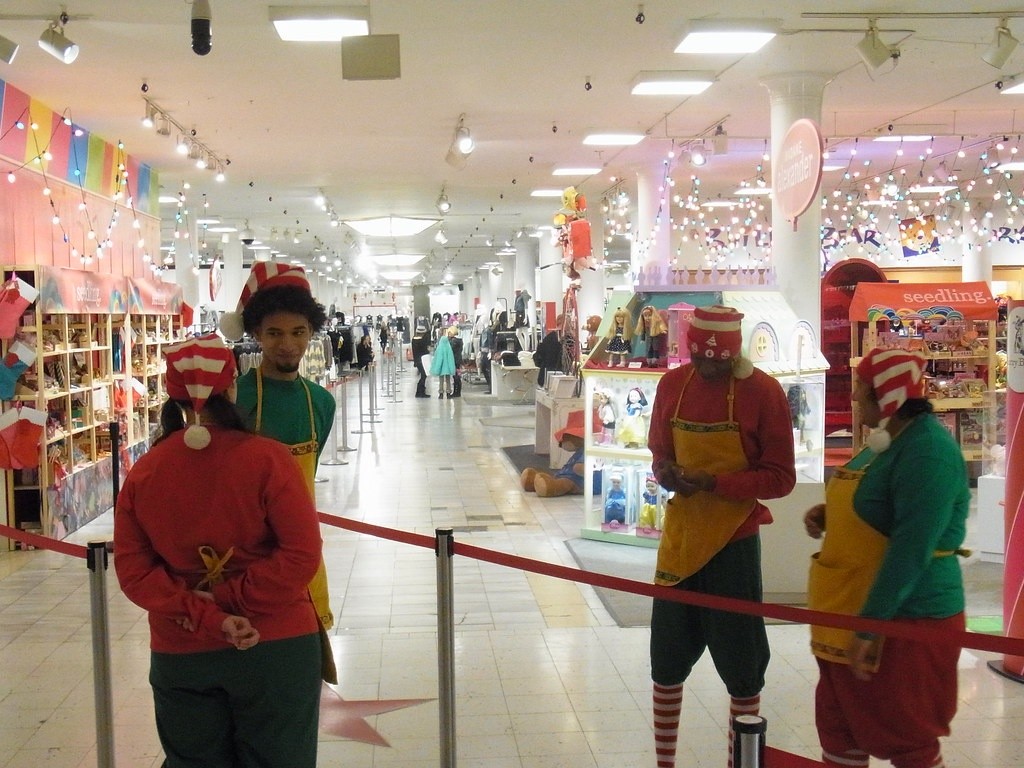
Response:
[438,393,443,399]
[447,393,452,399]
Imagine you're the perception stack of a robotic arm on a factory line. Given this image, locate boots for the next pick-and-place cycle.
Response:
[415,385,431,397]
[450,375,462,397]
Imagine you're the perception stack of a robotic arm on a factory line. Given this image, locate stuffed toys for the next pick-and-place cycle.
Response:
[521,433,585,496]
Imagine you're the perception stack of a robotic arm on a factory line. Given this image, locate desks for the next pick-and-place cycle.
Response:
[490,359,541,401]
[534,391,586,469]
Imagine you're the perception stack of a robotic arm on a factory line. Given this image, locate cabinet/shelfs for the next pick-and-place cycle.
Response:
[851,320,998,479]
[579,366,675,545]
[0,265,186,550]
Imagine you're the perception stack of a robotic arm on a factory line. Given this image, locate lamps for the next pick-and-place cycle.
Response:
[141,104,473,288]
[267,5,370,43]
[481,12,1017,273]
[0,33,18,63]
[38,14,79,64]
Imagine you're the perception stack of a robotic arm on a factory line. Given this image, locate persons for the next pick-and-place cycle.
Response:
[514,290,524,325]
[355,312,451,332]
[378,322,388,354]
[412,327,431,397]
[606,476,627,524]
[789,387,810,430]
[595,391,616,446]
[636,305,668,368]
[616,388,649,449]
[606,308,633,367]
[429,327,464,399]
[357,336,375,371]
[113,334,323,768]
[328,318,343,357]
[222,262,338,685]
[804,348,971,768]
[641,477,665,533]
[647,306,796,768]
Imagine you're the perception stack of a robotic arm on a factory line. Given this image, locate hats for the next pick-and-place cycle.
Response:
[686,306,754,380]
[447,326,458,335]
[416,326,426,333]
[160,332,236,450]
[219,260,311,341]
[856,347,928,453]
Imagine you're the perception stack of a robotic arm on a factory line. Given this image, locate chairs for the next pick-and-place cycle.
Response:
[514,370,540,406]
